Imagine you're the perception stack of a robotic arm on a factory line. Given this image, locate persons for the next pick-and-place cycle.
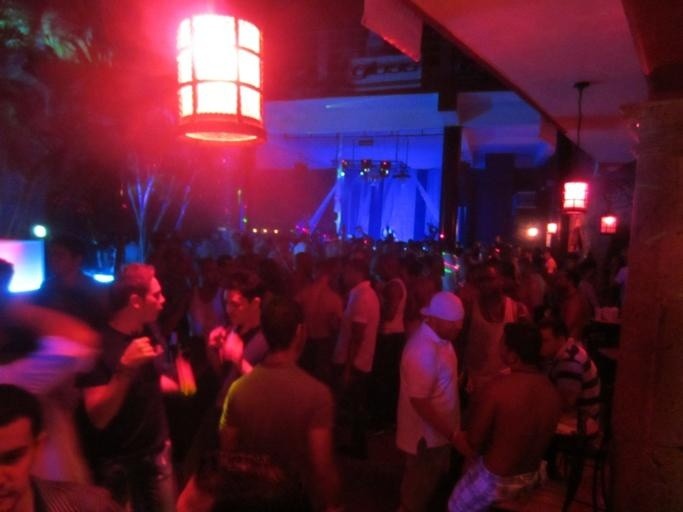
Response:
[1,224,632,512]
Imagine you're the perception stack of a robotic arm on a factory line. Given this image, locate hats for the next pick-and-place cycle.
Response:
[419,290,465,322]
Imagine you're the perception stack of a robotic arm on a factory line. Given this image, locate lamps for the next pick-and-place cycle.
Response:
[562,81,591,216]
[174,8,264,149]
[600,213,618,234]
